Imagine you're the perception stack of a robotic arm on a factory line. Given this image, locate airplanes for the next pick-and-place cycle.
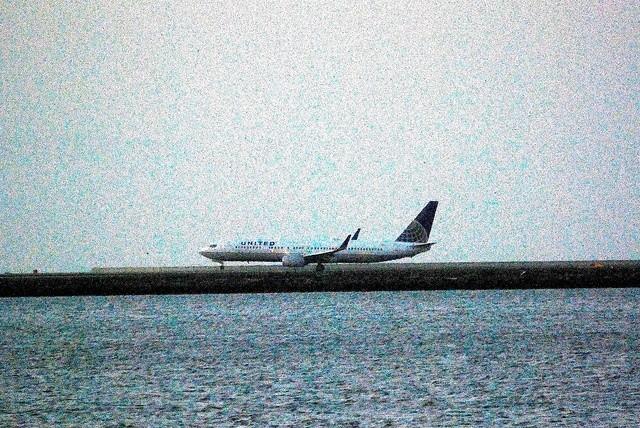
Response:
[199,201,437,271]
